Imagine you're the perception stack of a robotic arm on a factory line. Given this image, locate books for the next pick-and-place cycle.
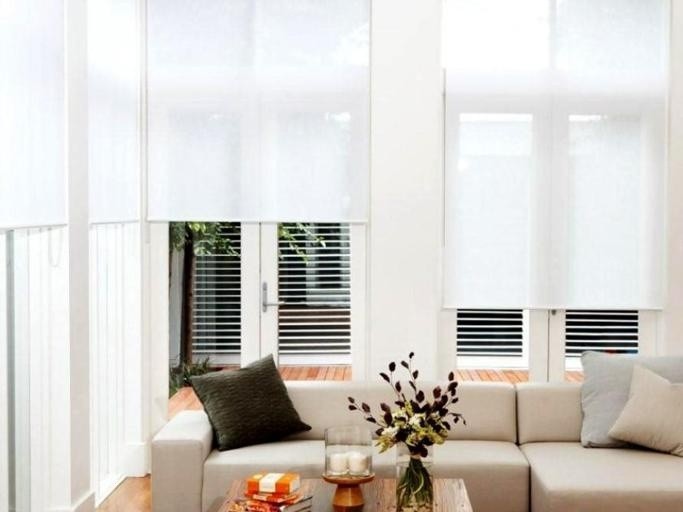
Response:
[227,498,314,512]
[247,470,300,494]
[243,491,297,504]
[282,495,305,505]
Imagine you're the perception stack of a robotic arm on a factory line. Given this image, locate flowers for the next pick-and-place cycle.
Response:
[348,351,469,504]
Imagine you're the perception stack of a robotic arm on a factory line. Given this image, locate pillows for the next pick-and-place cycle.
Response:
[188,354,313,452]
[576,349,683,460]
